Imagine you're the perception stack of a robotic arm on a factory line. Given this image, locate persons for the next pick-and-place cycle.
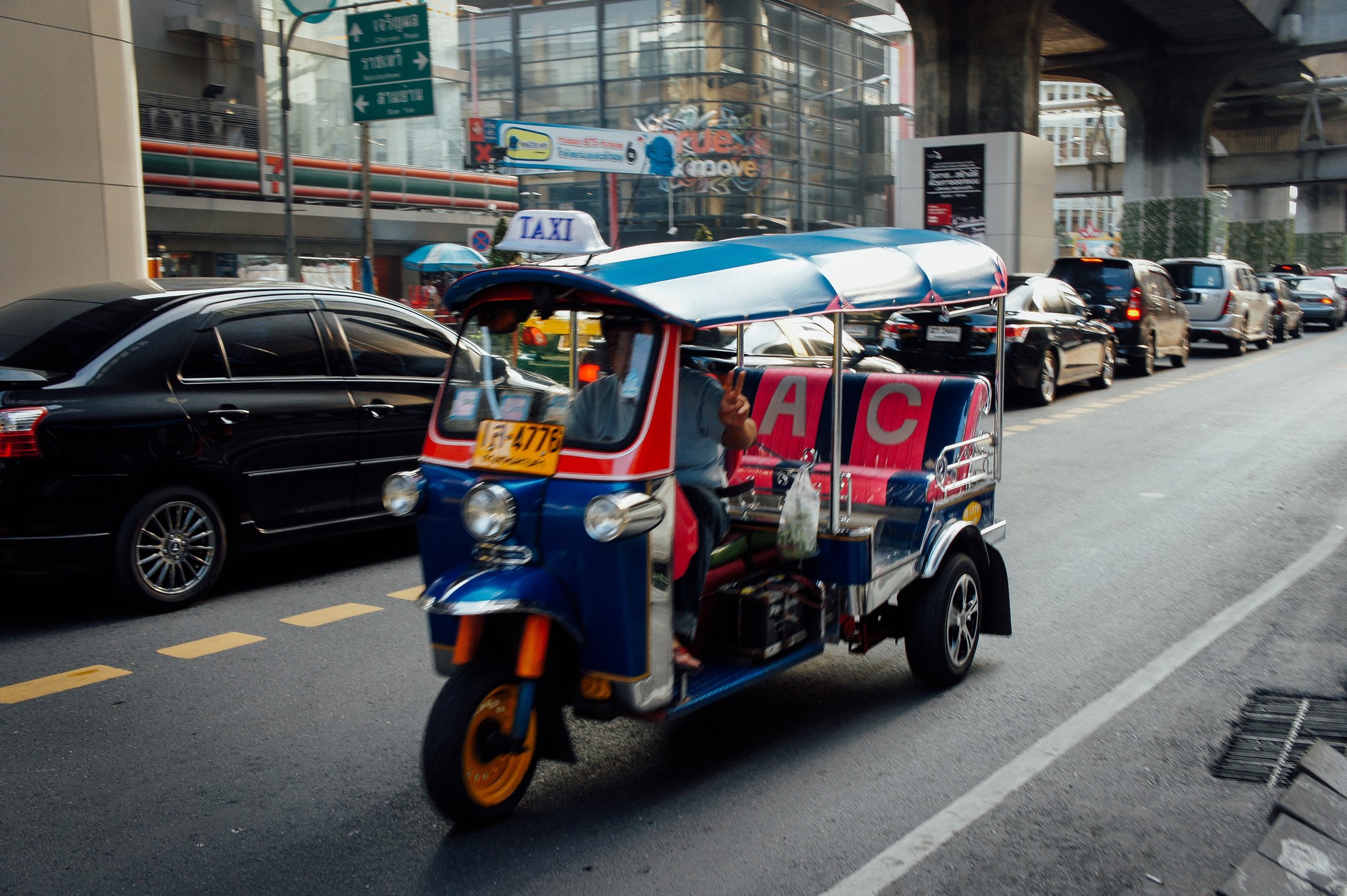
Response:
[544,309,758,672]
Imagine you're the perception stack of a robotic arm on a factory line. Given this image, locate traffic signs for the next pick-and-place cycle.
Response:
[349,39,433,88]
[349,78,434,123]
[345,2,428,51]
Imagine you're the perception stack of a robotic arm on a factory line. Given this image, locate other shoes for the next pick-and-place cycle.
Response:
[672,637,700,671]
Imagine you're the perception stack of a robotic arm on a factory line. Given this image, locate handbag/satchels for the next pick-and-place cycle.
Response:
[777,464,820,558]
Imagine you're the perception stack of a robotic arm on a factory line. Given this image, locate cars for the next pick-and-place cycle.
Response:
[1255,263,1347,344]
[874,271,1118,406]
[578,314,905,391]
[511,309,606,388]
[0,278,573,616]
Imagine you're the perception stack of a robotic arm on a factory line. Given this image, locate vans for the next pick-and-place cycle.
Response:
[1046,254,1192,377]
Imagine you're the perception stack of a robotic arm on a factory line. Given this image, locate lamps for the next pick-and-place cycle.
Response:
[202,82,226,100]
[492,144,509,161]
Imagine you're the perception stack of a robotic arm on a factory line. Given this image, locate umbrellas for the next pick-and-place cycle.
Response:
[401,244,490,272]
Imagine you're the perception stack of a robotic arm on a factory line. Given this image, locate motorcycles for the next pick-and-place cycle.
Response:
[380,209,1015,833]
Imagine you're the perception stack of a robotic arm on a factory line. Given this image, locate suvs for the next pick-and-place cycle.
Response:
[1156,258,1276,357]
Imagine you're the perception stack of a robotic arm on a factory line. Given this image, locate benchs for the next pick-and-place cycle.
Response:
[726,467,887,579]
[722,365,986,540]
